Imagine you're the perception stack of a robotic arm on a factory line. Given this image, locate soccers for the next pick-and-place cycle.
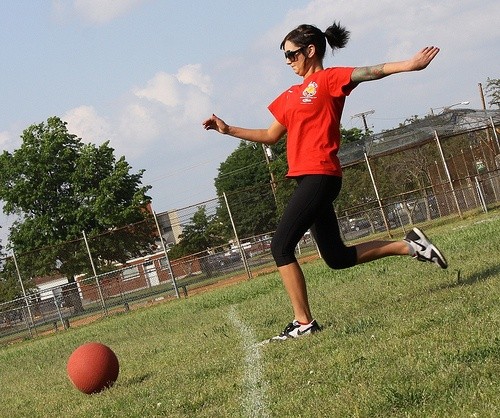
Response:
[66,342,120,395]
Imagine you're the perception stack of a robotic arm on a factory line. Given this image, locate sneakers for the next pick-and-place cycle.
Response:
[270,316,320,341]
[403,227,449,269]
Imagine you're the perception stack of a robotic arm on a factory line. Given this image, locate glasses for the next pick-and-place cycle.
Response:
[284,47,308,63]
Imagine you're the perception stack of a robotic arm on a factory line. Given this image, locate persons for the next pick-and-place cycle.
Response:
[202,20,448,342]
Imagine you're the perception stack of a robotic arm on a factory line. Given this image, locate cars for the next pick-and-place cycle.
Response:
[208,196,438,268]
